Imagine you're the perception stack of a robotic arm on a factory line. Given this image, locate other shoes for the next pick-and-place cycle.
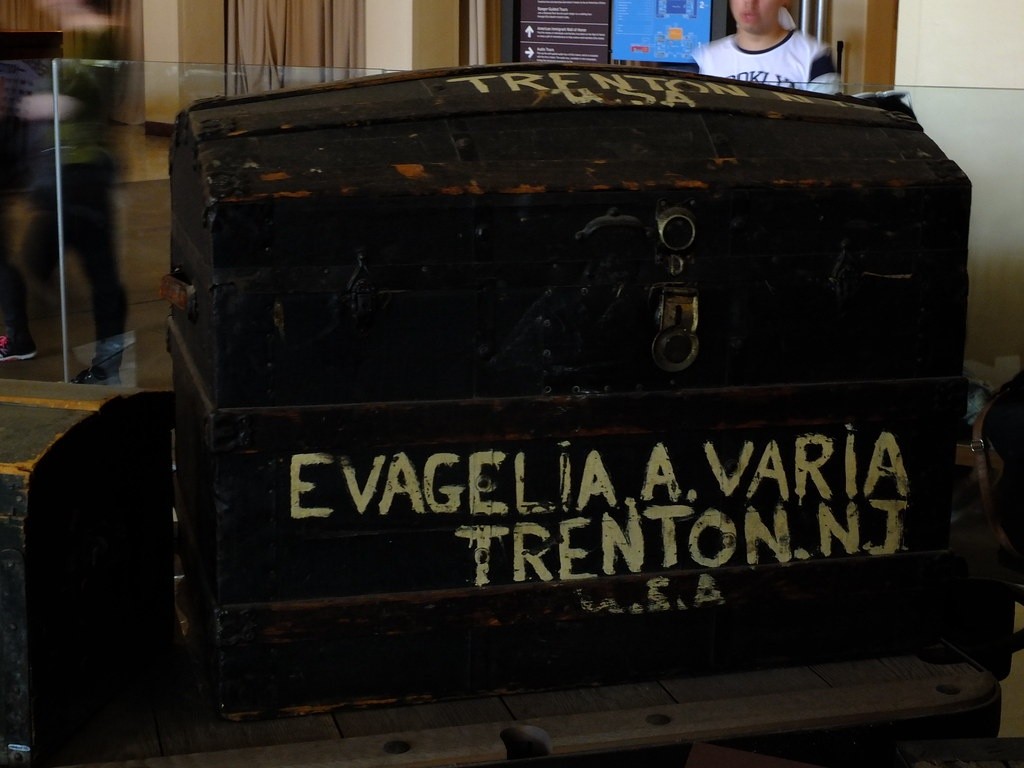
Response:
[58,367,122,387]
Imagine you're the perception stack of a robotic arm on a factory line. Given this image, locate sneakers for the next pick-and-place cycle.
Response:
[0,329,39,365]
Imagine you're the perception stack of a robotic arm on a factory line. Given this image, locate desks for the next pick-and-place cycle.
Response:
[51,638,1003,768]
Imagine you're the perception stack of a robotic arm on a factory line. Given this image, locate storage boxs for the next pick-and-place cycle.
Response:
[164,60,975,721]
[0,379,164,768]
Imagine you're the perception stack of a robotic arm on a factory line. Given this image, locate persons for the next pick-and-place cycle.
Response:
[0,1,126,384]
[690,0,844,95]
